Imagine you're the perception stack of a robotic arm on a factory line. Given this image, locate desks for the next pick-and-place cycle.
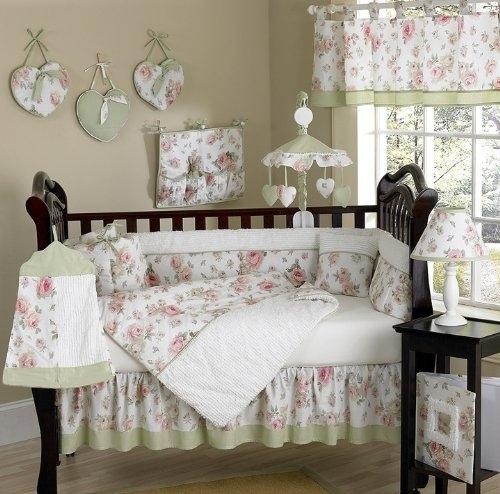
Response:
[391,311,500,494]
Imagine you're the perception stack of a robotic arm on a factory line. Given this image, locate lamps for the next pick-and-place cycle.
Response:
[410,208,490,326]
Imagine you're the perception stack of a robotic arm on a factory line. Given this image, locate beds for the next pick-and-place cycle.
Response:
[26,164,438,494]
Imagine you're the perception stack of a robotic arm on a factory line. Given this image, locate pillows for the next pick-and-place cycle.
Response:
[314,233,379,298]
[368,231,413,322]
